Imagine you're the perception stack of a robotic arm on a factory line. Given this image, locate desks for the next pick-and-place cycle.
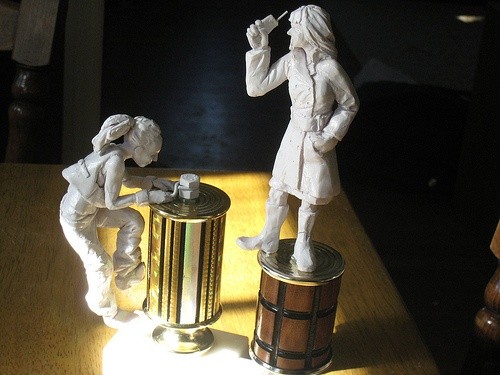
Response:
[0,162,440,375]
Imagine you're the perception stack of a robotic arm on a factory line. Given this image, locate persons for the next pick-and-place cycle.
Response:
[236,5,361,272]
[58,114,175,328]
